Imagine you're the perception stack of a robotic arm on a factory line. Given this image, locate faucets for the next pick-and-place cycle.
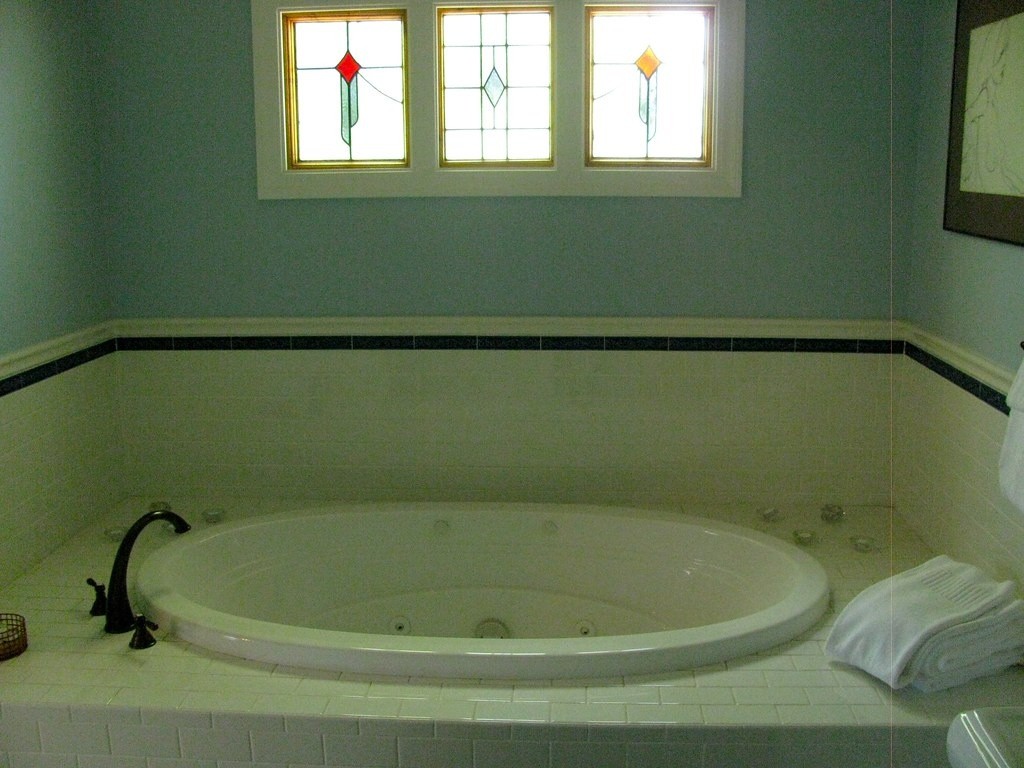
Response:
[103,509,191,634]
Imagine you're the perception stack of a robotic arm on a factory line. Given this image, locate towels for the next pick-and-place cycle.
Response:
[995,356,1023,512]
[824,553,1024,690]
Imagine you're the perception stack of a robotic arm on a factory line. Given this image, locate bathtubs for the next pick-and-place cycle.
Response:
[136,495,827,677]
